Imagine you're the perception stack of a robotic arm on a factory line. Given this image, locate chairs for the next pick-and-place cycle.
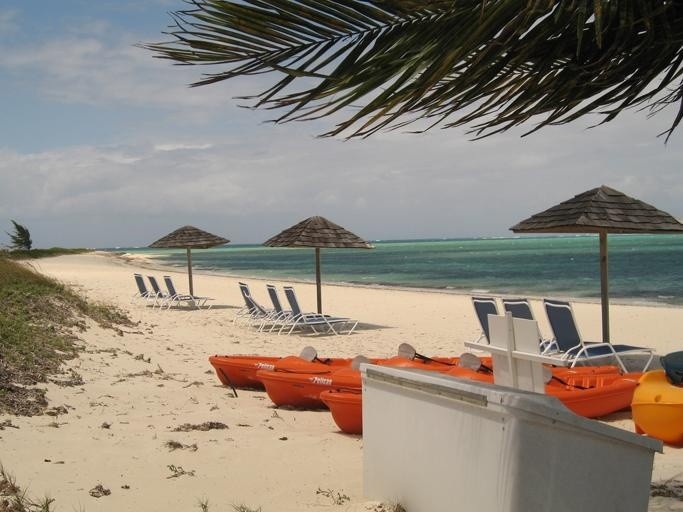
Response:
[232,279,371,339]
[462,286,662,375]
[127,271,223,313]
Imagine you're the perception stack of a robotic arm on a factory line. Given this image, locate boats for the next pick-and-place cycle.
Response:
[201,346,483,387]
[316,370,646,439]
[254,357,623,410]
[631,352,682,445]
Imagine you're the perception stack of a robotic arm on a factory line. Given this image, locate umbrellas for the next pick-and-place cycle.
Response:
[148,224,230,297]
[263,215,371,315]
[509,184,682,344]
